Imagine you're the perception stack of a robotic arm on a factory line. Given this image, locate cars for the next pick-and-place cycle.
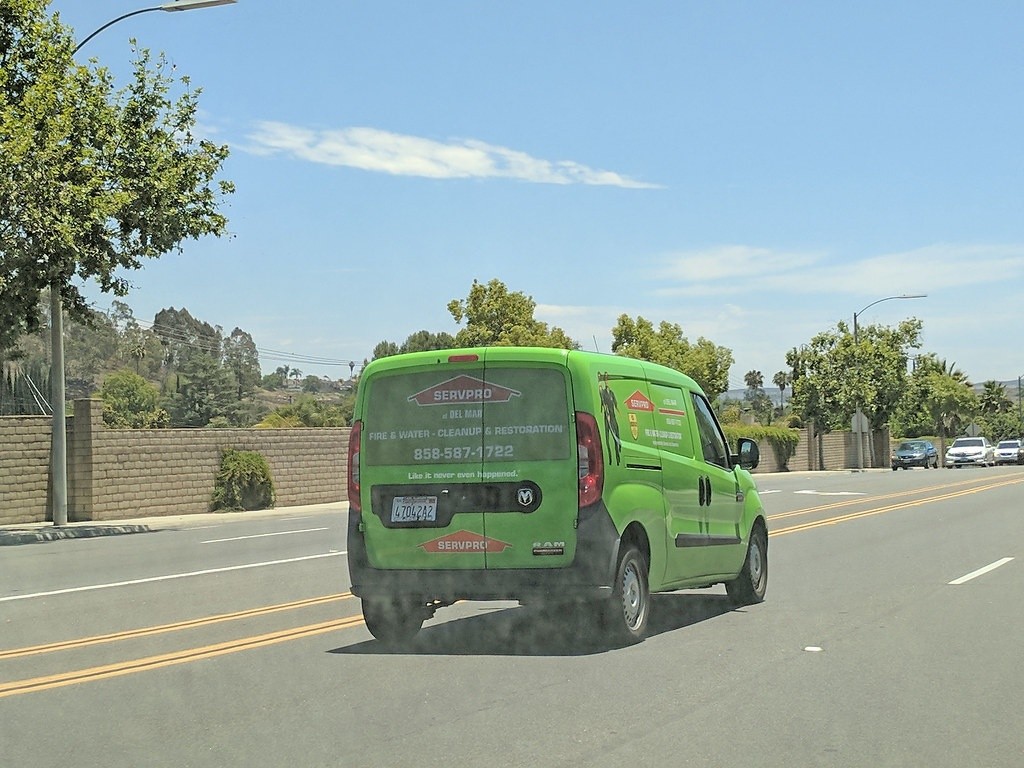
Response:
[892,441,938,470]
[944,437,994,469]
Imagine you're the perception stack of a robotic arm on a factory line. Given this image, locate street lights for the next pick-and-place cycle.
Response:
[854,294,927,469]
[51,0,238,524]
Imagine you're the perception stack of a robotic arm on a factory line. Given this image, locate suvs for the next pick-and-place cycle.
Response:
[992,441,1023,466]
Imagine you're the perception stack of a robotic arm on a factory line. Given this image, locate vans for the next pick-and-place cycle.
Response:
[347,346,770,644]
